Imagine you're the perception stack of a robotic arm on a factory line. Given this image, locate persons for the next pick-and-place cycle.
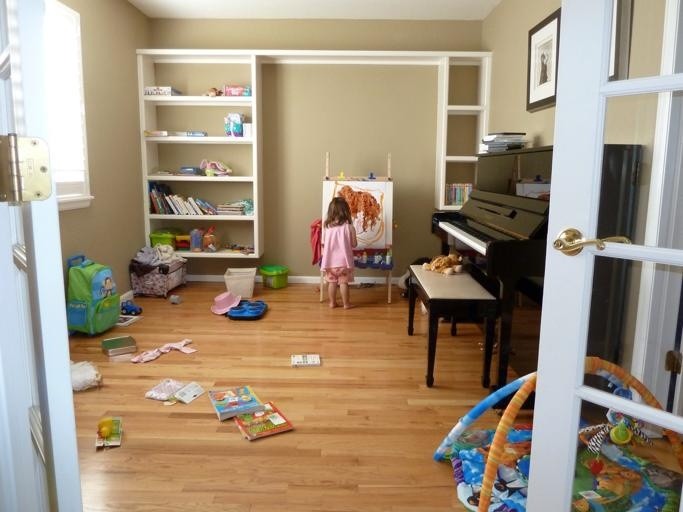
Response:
[320,197,357,309]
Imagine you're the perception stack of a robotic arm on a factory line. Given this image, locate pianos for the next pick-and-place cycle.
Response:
[431,144,642,410]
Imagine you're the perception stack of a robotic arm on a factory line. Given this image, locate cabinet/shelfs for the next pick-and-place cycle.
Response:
[135,48,265,260]
[433,51,492,212]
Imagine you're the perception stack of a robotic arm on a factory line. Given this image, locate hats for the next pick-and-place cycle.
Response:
[210,292,240,316]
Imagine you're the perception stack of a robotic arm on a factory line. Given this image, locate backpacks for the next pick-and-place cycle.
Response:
[68,254,119,335]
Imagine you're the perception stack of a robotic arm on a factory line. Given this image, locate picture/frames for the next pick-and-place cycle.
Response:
[525,1,632,113]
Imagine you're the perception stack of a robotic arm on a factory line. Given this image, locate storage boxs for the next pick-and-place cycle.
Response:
[129,258,187,298]
[148,227,181,251]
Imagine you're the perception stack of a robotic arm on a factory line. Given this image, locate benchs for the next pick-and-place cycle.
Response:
[406,264,497,386]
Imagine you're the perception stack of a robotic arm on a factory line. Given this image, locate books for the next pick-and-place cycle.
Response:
[232,401,293,441]
[208,384,266,422]
[445,183,473,205]
[477,131,526,153]
[149,183,246,216]
